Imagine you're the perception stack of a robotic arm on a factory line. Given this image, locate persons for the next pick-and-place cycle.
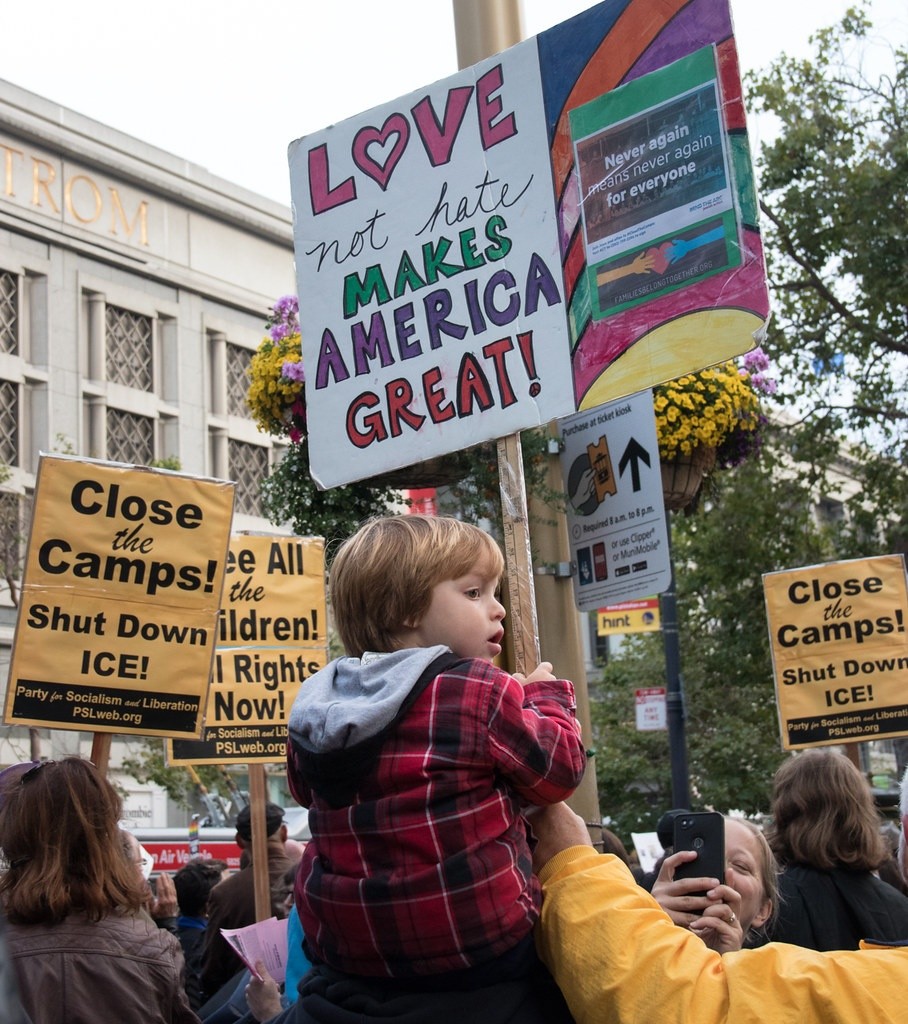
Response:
[601,828,645,884]
[0,758,311,1024]
[260,938,576,1024]
[529,767,908,1024]
[640,808,688,894]
[289,516,574,1023]
[762,746,908,952]
[652,817,782,955]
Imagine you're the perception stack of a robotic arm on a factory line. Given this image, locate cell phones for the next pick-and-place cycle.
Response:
[674,813,725,916]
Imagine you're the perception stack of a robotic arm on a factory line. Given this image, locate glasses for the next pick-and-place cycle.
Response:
[0,757,97,789]
[280,888,294,900]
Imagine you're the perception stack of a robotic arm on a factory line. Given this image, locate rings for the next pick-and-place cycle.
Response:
[726,913,736,923]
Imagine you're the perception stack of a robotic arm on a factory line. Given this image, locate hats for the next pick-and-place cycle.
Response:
[237,803,285,841]
[657,809,690,848]
[873,792,901,818]
